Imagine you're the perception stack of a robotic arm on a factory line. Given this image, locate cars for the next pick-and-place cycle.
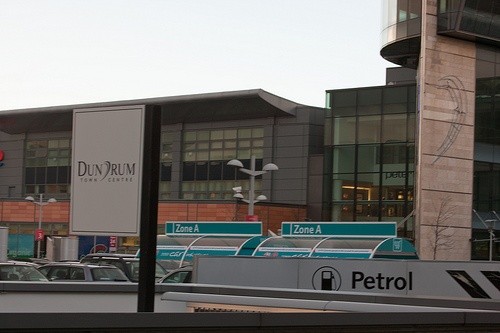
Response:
[1,253,192,284]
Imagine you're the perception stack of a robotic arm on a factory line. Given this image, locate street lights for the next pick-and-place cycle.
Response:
[227,156,278,221]
[25,193,57,259]
